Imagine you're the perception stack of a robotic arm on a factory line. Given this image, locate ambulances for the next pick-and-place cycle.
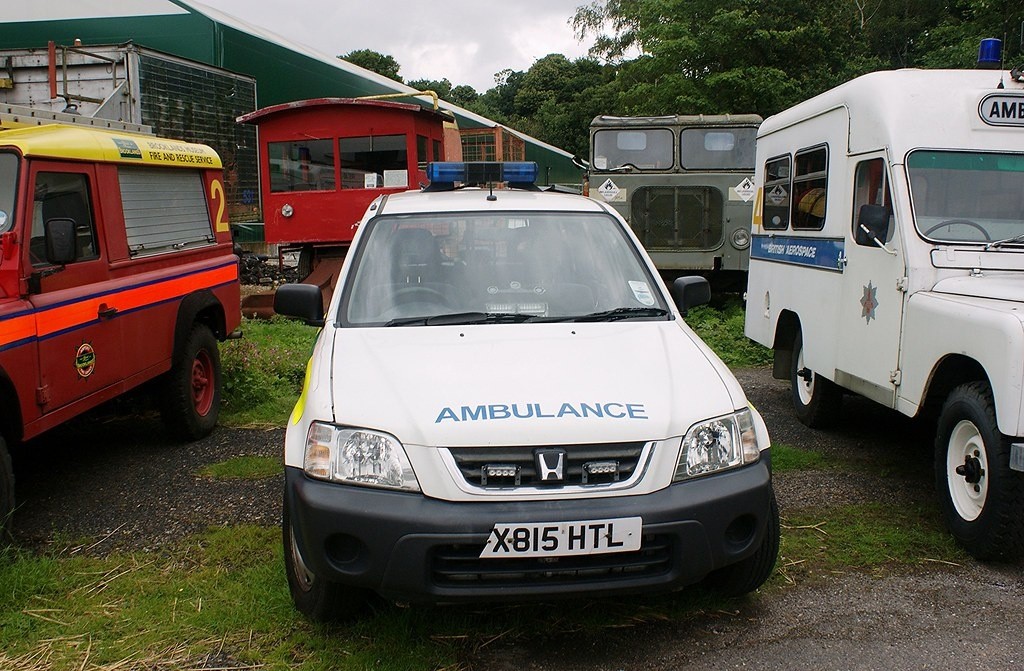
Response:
[271,161,780,623]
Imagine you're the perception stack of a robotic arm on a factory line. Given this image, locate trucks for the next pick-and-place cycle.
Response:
[743,37,1024,566]
[572,114,763,310]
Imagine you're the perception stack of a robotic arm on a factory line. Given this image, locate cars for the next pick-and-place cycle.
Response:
[0,120,245,547]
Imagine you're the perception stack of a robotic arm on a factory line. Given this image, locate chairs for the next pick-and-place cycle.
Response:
[368,227,467,317]
[30,190,93,264]
[503,234,606,315]
